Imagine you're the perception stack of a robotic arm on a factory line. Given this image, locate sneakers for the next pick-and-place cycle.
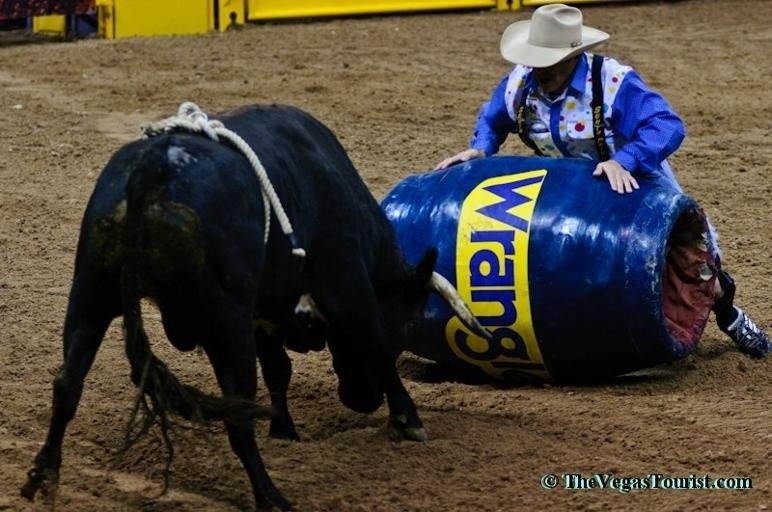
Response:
[717,305,771,359]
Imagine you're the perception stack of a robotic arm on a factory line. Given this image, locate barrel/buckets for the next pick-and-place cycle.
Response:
[379,156,717,387]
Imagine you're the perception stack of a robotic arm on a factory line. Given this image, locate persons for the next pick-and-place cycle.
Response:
[432,4,772,360]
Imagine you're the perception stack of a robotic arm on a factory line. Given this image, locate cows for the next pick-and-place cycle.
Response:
[21,104,494,512]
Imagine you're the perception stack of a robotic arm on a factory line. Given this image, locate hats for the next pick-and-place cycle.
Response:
[500,3,610,69]
